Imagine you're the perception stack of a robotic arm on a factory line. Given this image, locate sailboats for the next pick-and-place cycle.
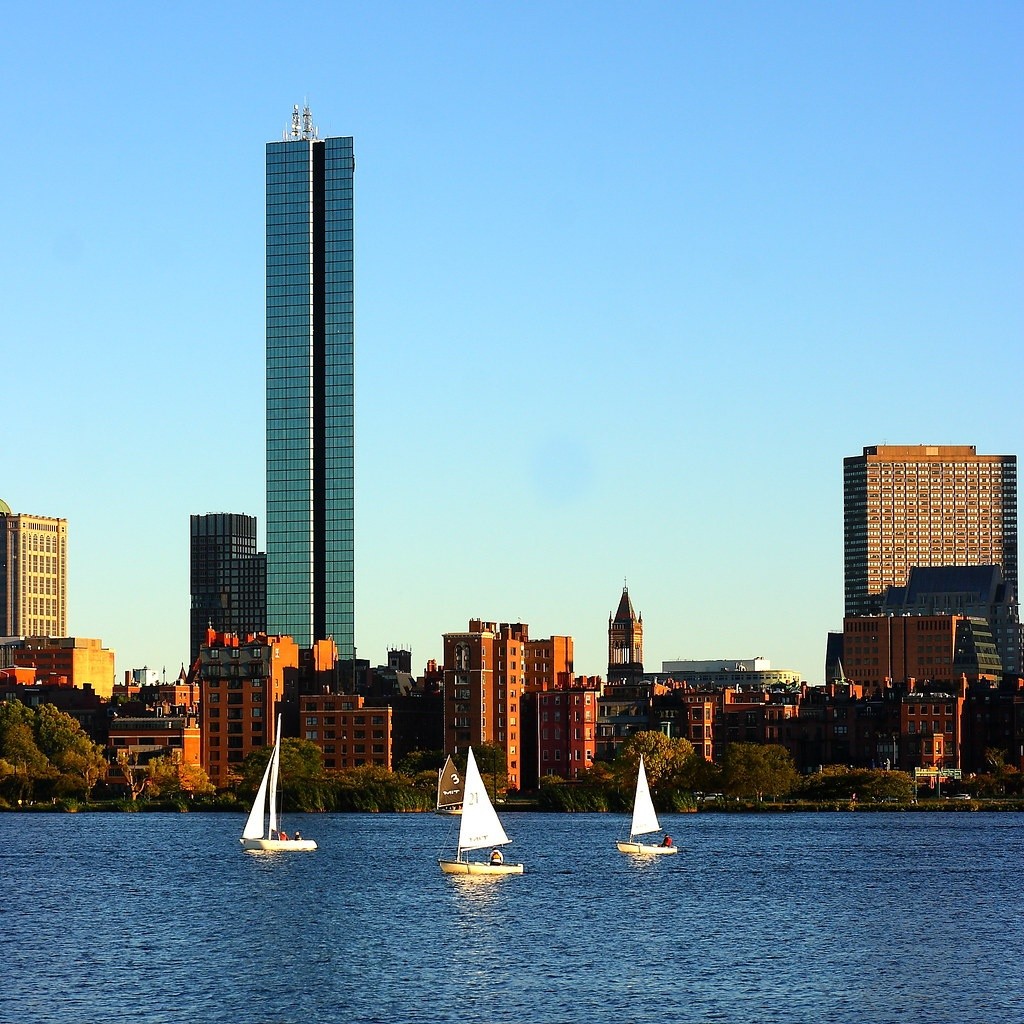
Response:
[240,712,318,854]
[436,745,525,878]
[435,754,465,815]
[615,754,678,855]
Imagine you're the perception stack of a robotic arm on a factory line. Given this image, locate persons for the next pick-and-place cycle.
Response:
[280,831,289,841]
[453,806,457,810]
[661,834,672,847]
[489,847,504,866]
[295,832,303,840]
